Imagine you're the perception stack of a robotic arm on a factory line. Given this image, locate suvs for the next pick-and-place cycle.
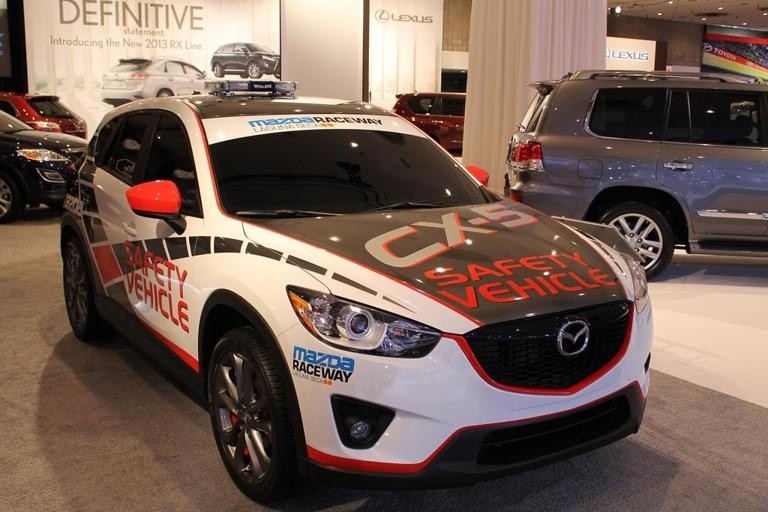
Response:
[390,92,466,153]
[495,68,768,281]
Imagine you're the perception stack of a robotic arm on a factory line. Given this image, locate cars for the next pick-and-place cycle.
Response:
[59,96,654,510]
[95,43,280,108]
[0,91,88,223]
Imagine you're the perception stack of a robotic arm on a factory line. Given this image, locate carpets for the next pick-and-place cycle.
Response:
[0,218,768,512]
